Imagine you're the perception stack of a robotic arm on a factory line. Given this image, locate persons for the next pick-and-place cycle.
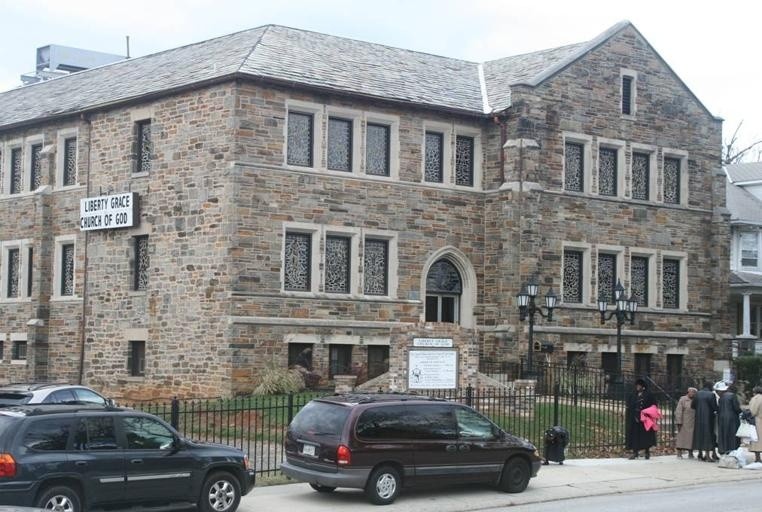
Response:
[626,380,657,459]
[673,380,762,464]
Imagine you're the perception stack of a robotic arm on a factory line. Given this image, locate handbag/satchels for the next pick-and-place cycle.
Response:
[741,424,758,442]
[735,423,752,438]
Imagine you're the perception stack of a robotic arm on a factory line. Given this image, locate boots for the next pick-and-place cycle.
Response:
[644,449,651,459]
[627,450,639,460]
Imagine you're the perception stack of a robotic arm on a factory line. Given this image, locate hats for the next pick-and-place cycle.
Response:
[713,381,728,391]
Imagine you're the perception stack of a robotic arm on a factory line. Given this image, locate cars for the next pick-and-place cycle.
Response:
[0,381,255,512]
[280,392,541,505]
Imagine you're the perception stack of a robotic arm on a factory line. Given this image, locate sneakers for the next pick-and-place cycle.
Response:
[674,448,722,463]
[541,458,564,465]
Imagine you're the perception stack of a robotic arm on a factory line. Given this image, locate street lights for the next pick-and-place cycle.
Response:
[515,274,555,374]
[597,278,639,381]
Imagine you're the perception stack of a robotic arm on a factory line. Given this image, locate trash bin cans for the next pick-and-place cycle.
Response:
[613,379,624,401]
